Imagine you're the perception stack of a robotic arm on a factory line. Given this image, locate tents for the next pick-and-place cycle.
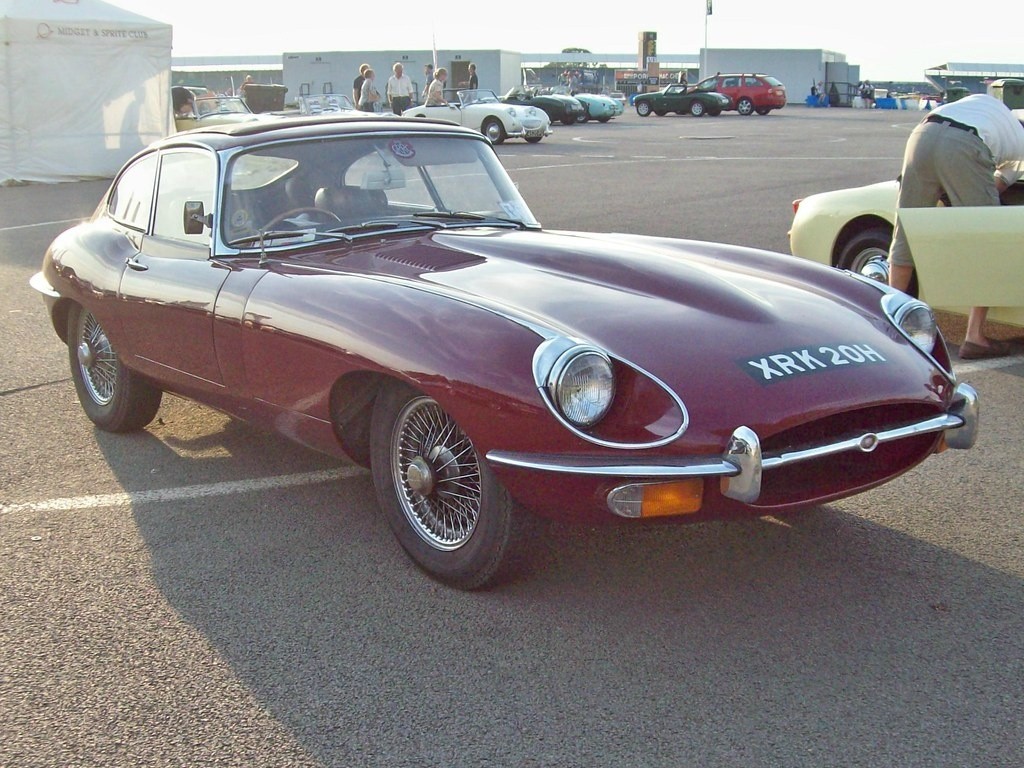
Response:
[0,0,179,185]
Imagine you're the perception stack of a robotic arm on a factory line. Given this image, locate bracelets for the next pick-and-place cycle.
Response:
[411,99,413,101]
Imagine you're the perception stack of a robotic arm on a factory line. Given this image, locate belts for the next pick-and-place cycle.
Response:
[928,115,979,136]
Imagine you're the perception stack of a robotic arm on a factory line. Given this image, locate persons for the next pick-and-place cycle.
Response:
[422,64,434,98]
[568,71,577,97]
[679,72,687,84]
[240,75,254,89]
[425,68,448,107]
[459,64,478,89]
[353,64,381,112]
[887,93,1024,359]
[387,63,414,116]
[816,80,826,104]
[859,80,874,98]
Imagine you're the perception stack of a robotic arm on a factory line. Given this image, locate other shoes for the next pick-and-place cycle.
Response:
[958,336,1011,358]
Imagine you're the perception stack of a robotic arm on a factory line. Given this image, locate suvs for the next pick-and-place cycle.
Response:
[687,71,787,116]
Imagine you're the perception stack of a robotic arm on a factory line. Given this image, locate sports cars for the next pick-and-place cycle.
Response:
[788,167,1024,328]
[633,83,731,117]
[28,109,981,592]
[400,88,554,146]
[476,83,625,126]
[173,86,376,132]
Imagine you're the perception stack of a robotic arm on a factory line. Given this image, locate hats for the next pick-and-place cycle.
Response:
[425,64,433,69]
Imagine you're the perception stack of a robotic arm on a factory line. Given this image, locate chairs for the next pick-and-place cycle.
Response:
[314,187,387,228]
[285,176,341,226]
